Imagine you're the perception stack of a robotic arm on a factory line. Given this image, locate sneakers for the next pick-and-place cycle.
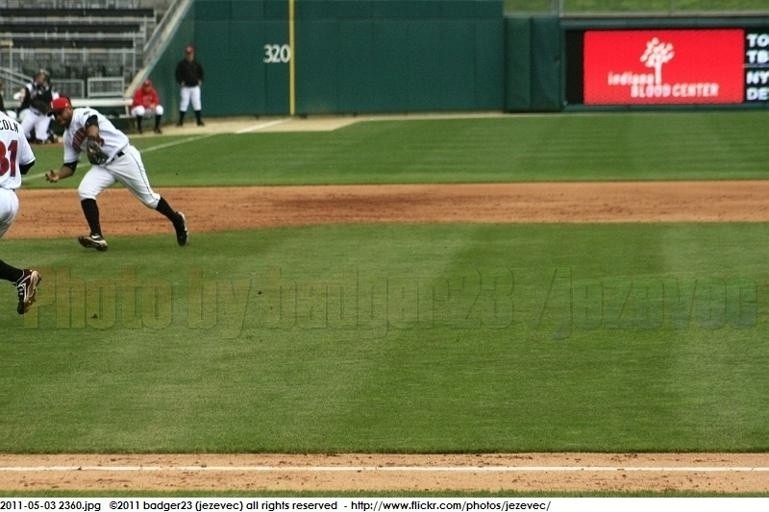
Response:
[79,234,107,250]
[173,212,187,245]
[13,270,41,314]
[137,120,204,134]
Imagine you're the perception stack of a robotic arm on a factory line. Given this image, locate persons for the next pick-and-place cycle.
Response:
[0,93,41,315]
[174,46,205,126]
[3,66,62,143]
[45,96,188,252]
[131,80,163,134]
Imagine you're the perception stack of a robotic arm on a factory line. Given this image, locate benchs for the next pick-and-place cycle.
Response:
[0,0,176,133]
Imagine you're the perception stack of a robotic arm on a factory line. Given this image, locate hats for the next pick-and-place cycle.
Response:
[144,80,151,86]
[185,46,193,54]
[47,97,71,117]
